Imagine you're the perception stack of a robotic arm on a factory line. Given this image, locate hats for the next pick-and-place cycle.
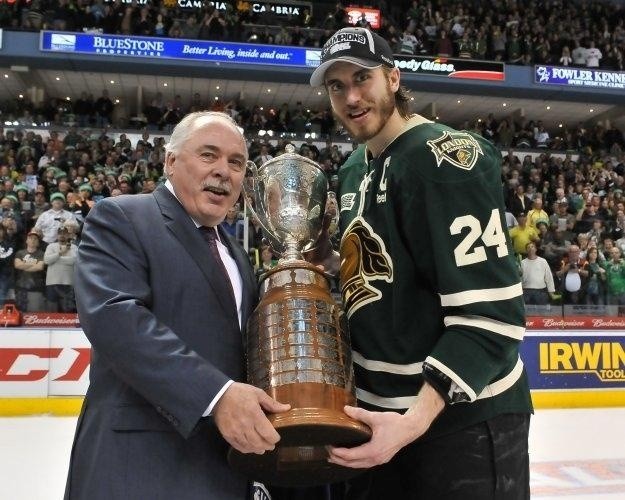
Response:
[56,225,69,233]
[310,25,397,89]
[27,231,41,238]
[0,127,160,210]
[516,158,623,235]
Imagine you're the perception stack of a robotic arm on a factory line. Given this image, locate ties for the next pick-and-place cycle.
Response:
[196,223,242,332]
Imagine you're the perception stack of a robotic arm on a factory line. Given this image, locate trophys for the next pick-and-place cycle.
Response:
[236,138,373,450]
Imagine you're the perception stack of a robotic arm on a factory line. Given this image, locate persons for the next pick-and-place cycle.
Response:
[306,27,537,500]
[60,110,296,500]
